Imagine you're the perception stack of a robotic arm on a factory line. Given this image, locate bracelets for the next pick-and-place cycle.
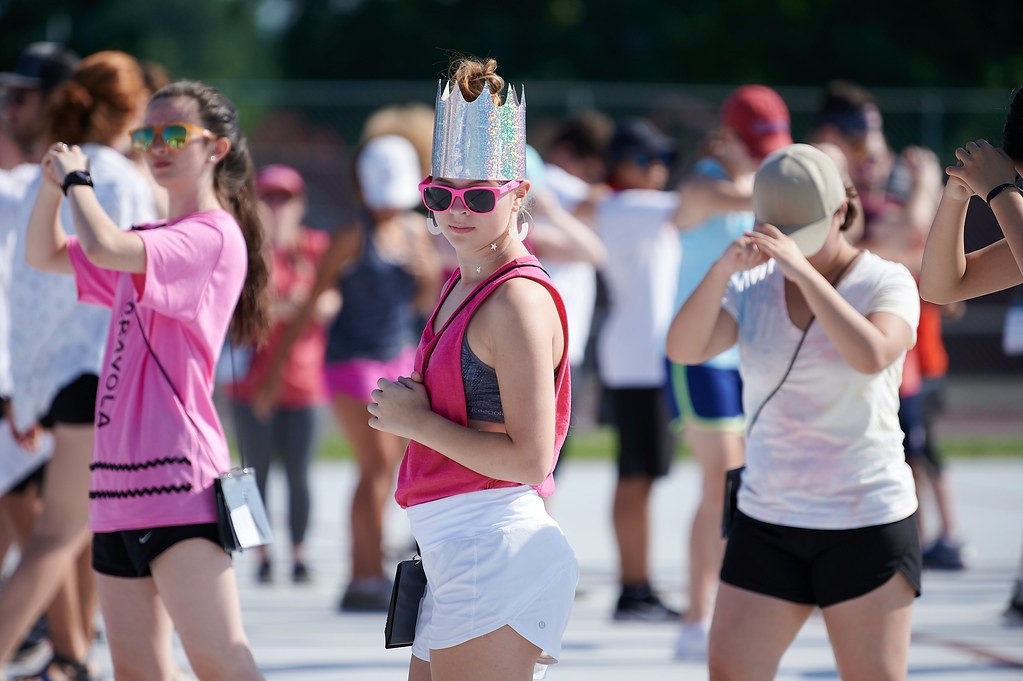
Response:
[986,183,1023,208]
[59,169,93,197]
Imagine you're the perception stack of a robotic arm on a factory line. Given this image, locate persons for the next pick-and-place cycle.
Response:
[0,38,1023,681]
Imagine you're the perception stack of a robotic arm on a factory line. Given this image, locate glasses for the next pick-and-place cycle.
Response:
[128,123,217,151]
[0,85,43,108]
[421,174,521,215]
[632,149,672,168]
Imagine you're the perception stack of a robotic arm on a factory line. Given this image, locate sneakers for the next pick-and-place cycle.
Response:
[614,582,682,622]
[293,562,307,582]
[260,558,274,581]
[340,580,393,612]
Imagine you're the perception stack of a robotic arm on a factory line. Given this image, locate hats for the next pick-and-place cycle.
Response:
[823,103,890,157]
[253,165,302,197]
[751,144,848,256]
[603,120,673,157]
[722,85,793,158]
[0,42,74,86]
[358,134,420,209]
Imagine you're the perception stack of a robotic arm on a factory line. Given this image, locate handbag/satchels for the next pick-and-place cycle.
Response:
[384,559,427,648]
[721,466,747,539]
[215,465,275,550]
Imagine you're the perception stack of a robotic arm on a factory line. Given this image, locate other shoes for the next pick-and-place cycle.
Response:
[1003,579,1023,626]
[675,623,710,659]
[920,537,965,571]
[41,652,110,681]
